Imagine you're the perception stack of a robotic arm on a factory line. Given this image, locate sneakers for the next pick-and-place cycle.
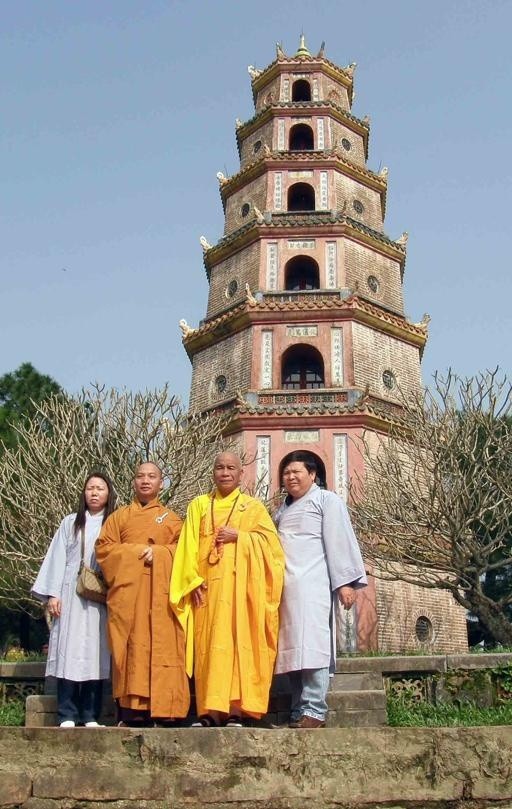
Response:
[149,719,173,728]
[83,721,105,729]
[59,720,75,728]
[118,719,134,727]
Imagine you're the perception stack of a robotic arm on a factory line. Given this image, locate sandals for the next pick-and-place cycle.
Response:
[191,715,220,726]
[224,715,247,727]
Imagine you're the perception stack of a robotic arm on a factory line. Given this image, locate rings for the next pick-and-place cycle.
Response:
[50,609,54,612]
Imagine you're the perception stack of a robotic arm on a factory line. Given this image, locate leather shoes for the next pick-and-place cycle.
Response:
[279,715,327,728]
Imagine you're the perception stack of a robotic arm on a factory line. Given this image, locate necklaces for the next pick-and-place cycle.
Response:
[208,493,239,534]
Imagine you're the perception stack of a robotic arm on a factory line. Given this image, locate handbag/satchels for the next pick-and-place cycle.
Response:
[76,567,107,604]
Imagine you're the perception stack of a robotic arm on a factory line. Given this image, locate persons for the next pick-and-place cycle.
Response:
[268,451,369,728]
[94,461,191,728]
[29,472,114,728]
[169,451,286,726]
